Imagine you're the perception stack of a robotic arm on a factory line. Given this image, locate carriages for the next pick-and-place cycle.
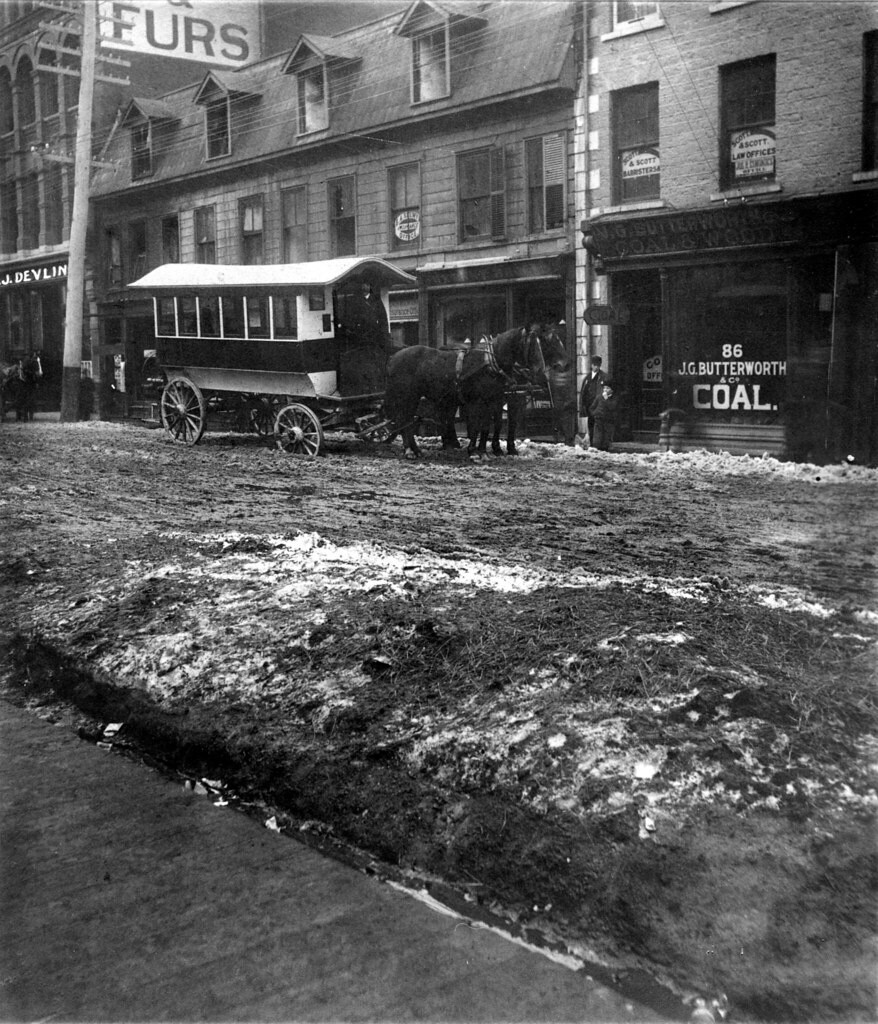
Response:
[127,253,571,459]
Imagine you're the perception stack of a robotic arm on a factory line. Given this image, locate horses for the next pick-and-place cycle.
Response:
[374,320,572,460]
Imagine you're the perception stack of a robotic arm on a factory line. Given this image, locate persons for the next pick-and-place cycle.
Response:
[588,381,619,451]
[579,355,610,447]
